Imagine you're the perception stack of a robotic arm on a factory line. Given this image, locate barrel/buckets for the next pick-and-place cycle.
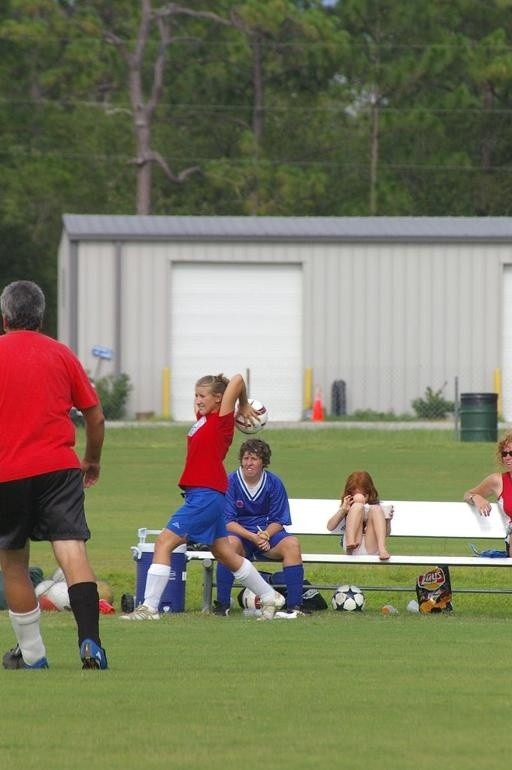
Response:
[458,392,498,442]
[135,542,186,613]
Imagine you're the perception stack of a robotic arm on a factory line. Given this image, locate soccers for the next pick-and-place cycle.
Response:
[33,567,113,612]
[331,585,366,611]
[234,399,268,434]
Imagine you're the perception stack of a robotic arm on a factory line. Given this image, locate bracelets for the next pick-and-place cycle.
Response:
[263,530,271,540]
[467,493,476,504]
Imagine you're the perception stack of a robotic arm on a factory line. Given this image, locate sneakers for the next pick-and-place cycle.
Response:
[80,639,107,669]
[3,643,50,670]
[281,605,312,617]
[117,602,160,621]
[257,592,286,623]
[212,600,231,616]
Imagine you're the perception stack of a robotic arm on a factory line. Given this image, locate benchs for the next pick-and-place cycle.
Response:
[186,497,512,615]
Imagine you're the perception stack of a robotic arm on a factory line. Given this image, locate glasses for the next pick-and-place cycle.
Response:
[501,451,512,458]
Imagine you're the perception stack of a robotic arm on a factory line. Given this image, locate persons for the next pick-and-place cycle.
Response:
[214,437,313,618]
[1,277,109,671]
[325,470,395,561]
[117,373,287,623]
[462,429,512,558]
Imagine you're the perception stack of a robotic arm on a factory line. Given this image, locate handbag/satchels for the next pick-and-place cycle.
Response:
[239,571,328,610]
